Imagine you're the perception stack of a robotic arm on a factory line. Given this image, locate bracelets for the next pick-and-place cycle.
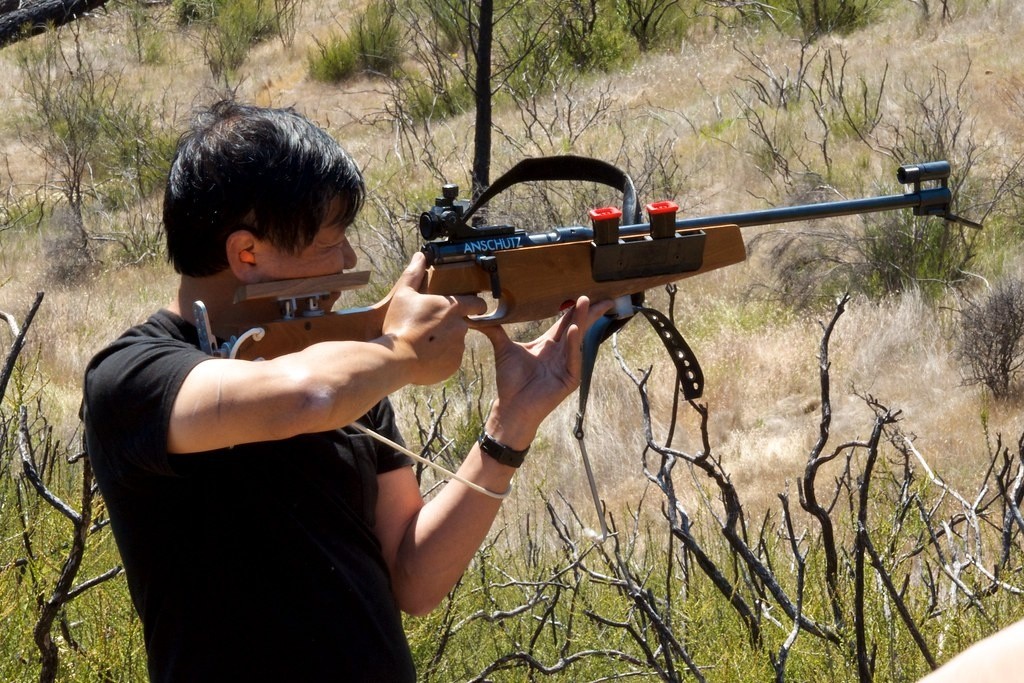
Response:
[477,425,530,468]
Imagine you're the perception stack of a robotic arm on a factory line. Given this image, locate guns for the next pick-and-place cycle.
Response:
[192,159,985,361]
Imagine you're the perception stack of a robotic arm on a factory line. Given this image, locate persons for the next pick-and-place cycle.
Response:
[84,99,615,683]
